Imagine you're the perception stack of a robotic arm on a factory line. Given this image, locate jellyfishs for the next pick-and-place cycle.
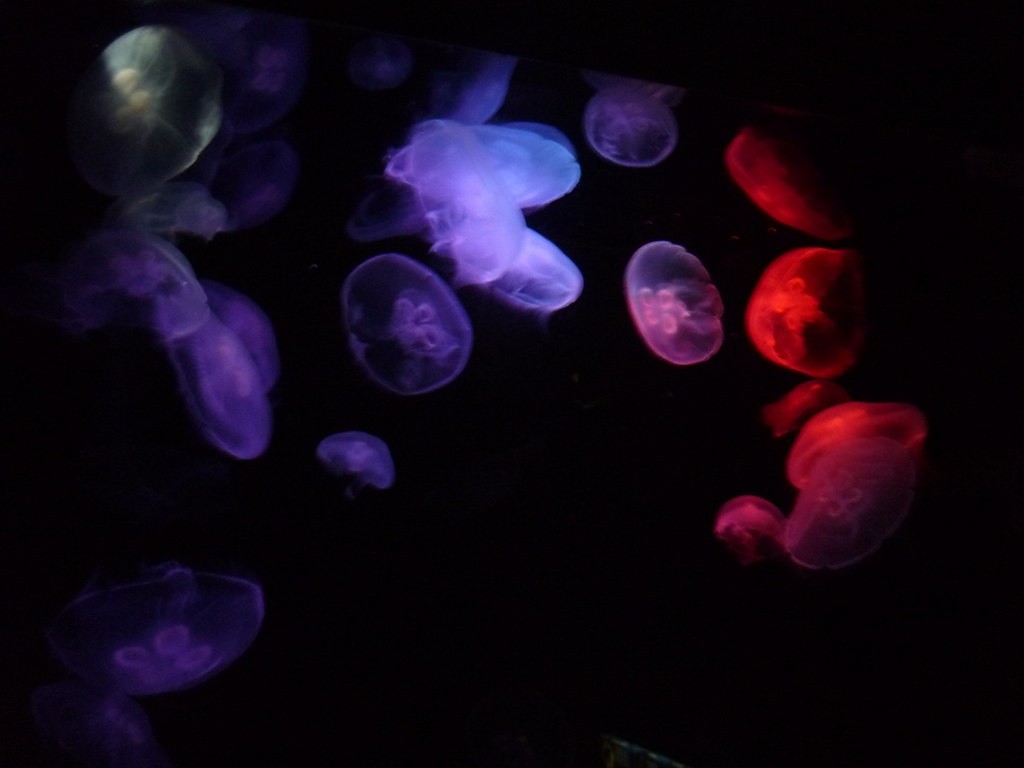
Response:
[33,1,924,761]
